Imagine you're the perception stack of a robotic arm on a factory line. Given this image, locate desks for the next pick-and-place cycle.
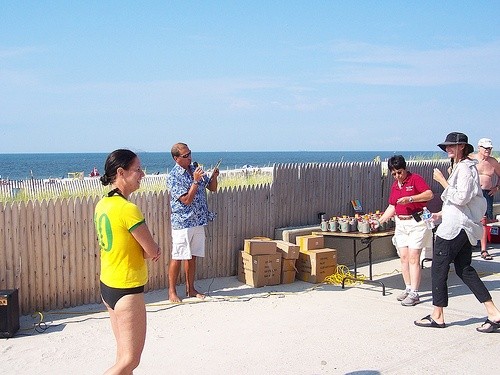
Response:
[311,224,440,296]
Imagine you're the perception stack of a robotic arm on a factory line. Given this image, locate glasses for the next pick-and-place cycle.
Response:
[392,172,401,175]
[182,150,192,159]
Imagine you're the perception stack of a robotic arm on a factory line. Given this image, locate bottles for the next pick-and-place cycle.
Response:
[423,207,435,230]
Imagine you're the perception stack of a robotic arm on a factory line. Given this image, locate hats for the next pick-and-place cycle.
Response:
[478,138,493,148]
[437,131,474,156]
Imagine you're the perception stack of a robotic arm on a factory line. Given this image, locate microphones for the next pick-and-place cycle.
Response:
[193,161,205,182]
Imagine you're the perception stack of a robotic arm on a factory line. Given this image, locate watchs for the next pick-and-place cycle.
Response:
[409,197,413,203]
[193,180,199,184]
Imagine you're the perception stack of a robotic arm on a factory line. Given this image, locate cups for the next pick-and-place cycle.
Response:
[320,213,393,233]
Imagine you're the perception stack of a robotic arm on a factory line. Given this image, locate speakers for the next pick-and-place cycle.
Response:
[0,289,20,338]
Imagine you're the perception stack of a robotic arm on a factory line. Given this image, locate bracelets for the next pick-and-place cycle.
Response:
[376,219,380,228]
[496,185,500,188]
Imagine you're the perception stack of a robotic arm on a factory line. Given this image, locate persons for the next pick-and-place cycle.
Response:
[370,155,432,306]
[414,132,500,333]
[447,138,500,220]
[0,174,1,179]
[166,143,219,302]
[94,149,162,375]
[90,168,99,176]
[156,170,159,175]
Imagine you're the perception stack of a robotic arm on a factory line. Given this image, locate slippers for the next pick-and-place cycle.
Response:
[414,315,444,328]
[476,318,500,332]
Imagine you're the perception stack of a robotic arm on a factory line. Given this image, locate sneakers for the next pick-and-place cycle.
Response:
[401,293,420,306]
[396,289,409,300]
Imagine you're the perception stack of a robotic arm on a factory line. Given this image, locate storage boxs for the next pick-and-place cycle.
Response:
[243,237,277,255]
[296,247,338,284]
[486,222,500,245]
[296,235,325,252]
[237,250,281,288]
[275,241,300,259]
[281,257,295,271]
[280,270,296,284]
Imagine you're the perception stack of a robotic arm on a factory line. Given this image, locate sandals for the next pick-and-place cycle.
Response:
[480,250,492,259]
